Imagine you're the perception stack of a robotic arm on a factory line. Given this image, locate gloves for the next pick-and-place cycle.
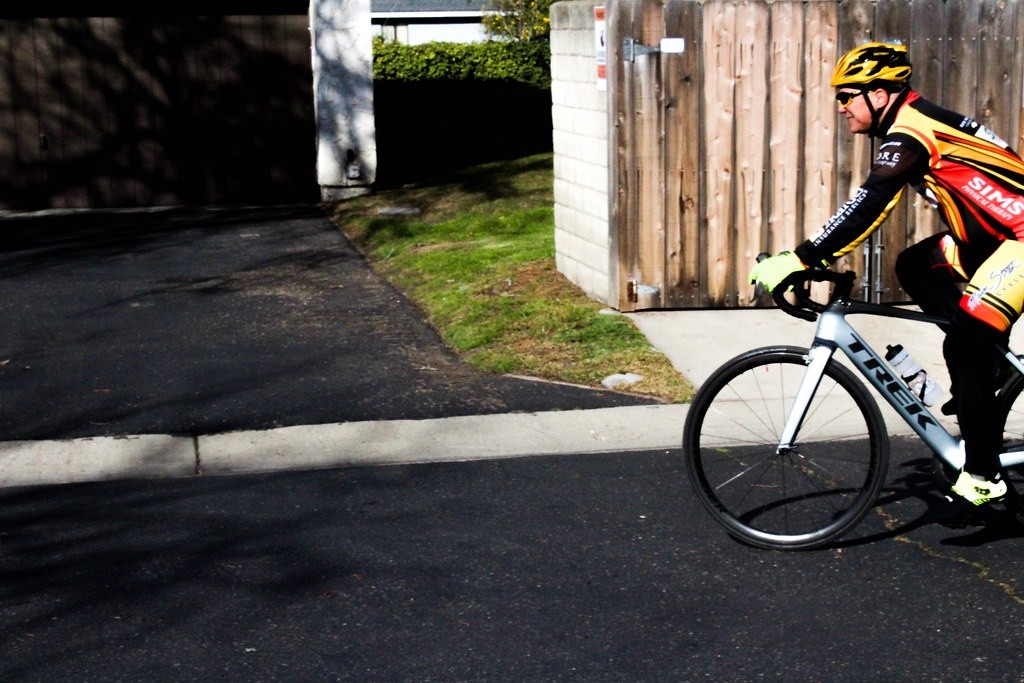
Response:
[748,252,806,292]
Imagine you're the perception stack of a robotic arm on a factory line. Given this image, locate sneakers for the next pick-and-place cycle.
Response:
[936,471,1007,512]
[941,398,956,415]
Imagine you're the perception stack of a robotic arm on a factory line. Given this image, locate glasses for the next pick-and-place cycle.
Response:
[835,91,863,108]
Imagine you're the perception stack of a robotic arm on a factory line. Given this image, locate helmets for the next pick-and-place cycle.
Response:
[830,42,913,93]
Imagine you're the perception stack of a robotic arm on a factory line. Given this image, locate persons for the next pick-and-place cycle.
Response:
[747,43,1024,521]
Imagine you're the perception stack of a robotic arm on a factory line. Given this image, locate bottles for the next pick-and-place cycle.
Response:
[884,344,945,406]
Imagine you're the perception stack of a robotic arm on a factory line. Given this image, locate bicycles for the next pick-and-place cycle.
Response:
[681,252,1024,552]
